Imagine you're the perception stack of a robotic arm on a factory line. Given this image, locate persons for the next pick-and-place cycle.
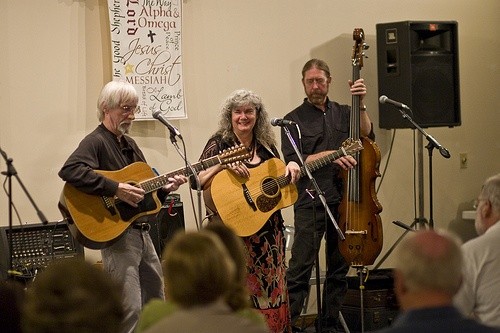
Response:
[389,231,500,333]
[22,260,130,333]
[135,230,269,333]
[189,90,300,333]
[281,60,375,333]
[58,80,187,333]
[205,222,246,291]
[453,176,500,327]
[0,280,25,333]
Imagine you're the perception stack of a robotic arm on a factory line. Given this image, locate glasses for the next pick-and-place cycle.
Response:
[473,198,492,208]
[117,104,140,113]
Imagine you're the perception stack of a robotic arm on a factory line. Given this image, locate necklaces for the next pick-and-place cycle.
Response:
[233,133,256,162]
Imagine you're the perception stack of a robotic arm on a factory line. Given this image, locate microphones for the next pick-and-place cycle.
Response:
[379,95,407,109]
[270,117,297,126]
[152,109,182,139]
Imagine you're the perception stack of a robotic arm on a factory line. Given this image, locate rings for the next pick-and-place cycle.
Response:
[135,198,138,202]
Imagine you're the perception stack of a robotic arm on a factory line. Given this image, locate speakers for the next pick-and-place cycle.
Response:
[150,204,184,259]
[376,20,461,128]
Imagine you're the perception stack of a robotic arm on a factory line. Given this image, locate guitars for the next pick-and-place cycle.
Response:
[201,138,364,238]
[58,141,250,249]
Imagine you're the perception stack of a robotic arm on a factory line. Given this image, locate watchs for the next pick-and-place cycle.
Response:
[360,105,366,111]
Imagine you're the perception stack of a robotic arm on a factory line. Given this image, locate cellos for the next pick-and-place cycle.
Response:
[336,29,382,333]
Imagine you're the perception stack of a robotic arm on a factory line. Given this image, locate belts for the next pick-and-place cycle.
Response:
[133,223,150,231]
